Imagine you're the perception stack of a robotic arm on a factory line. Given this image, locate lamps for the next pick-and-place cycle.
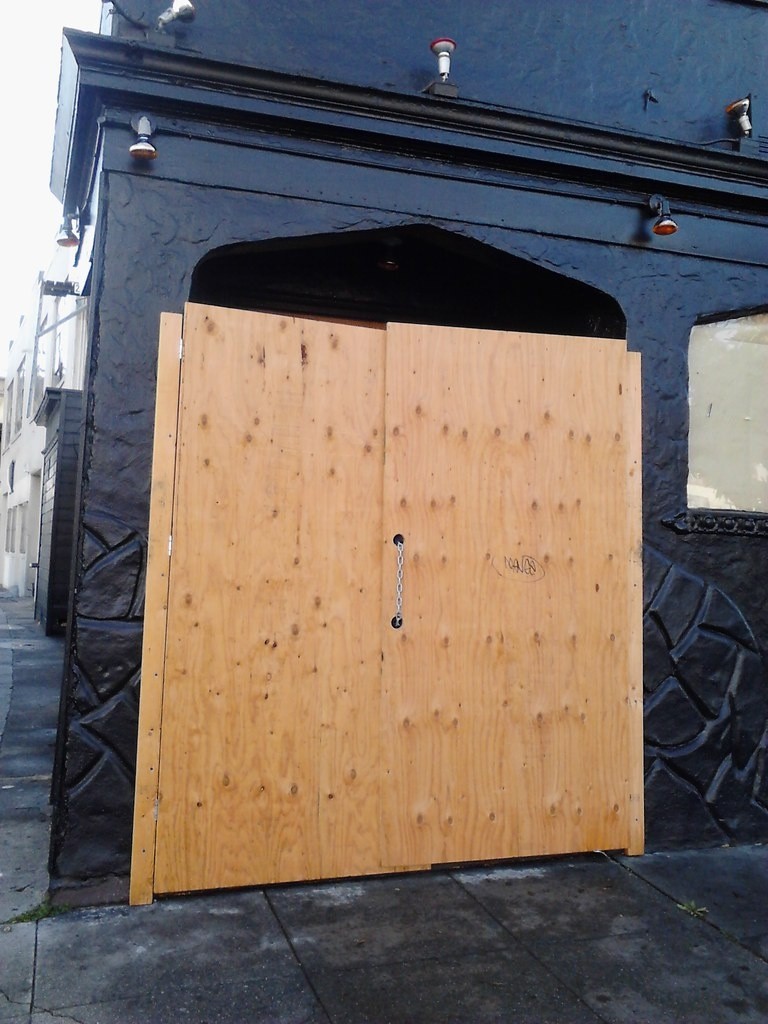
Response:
[649,191,679,236]
[128,110,159,161]
[699,97,753,145]
[56,206,87,246]
[419,38,461,98]
[144,0,197,48]
